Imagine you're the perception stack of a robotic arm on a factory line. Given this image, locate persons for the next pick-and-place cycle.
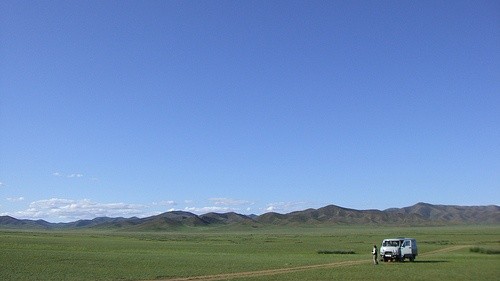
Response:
[371,245,378,265]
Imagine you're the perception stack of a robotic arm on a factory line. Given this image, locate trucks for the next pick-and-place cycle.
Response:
[380,237,418,263]
